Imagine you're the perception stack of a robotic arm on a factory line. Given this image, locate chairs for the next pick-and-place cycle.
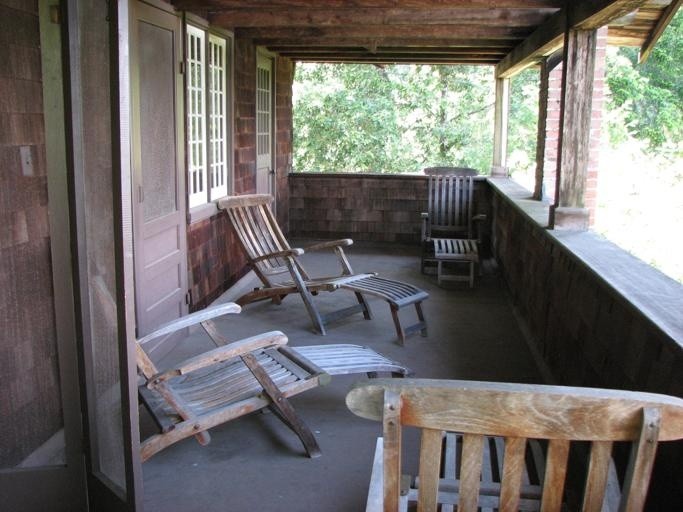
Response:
[345,375,683,512]
[89,273,420,477]
[418,166,491,292]
[217,191,431,348]
